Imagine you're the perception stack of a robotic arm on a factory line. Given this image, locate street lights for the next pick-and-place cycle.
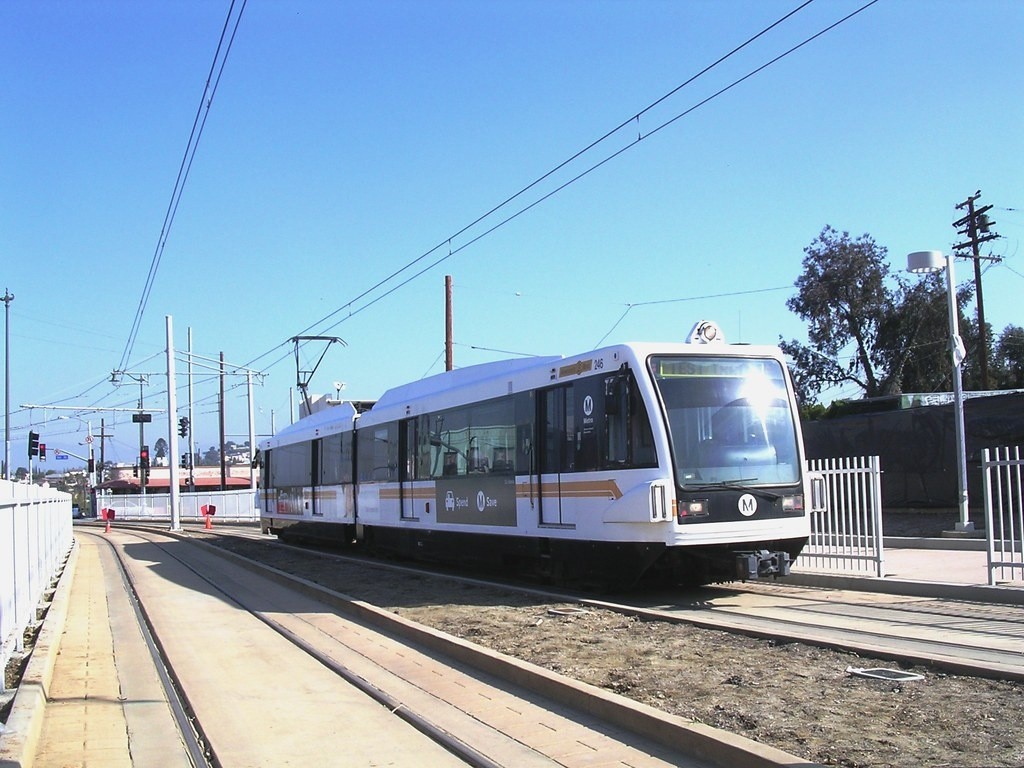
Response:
[907,248,969,531]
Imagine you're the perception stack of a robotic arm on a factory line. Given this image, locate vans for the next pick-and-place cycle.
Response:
[72,503,81,519]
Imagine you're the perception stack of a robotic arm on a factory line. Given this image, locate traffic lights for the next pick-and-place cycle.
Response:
[142,445,149,470]
[133,465,138,477]
[145,467,150,477]
[182,454,188,470]
[88,459,95,472]
[133,414,151,422]
[29,431,40,457]
[178,422,188,438]
[40,444,45,462]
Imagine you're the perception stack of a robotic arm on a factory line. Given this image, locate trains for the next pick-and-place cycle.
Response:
[250,321,811,589]
